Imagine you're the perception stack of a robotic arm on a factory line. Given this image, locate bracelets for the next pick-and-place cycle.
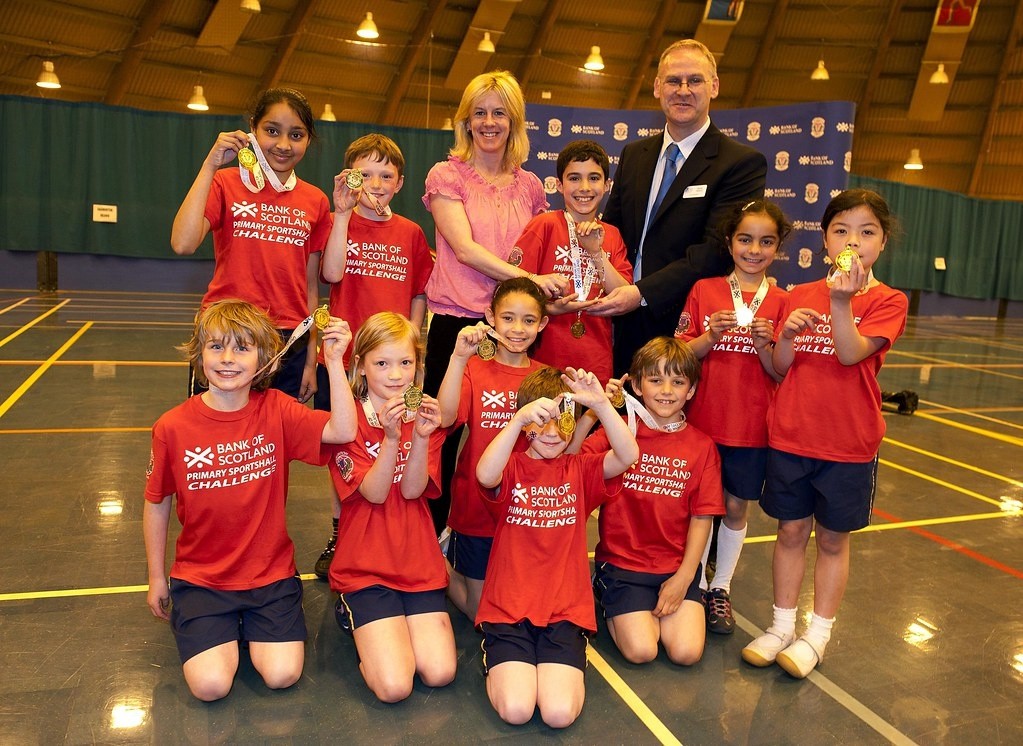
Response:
[526,271,538,283]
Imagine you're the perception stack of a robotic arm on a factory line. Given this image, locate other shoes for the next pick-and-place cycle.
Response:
[742,628,796,667]
[776,632,824,679]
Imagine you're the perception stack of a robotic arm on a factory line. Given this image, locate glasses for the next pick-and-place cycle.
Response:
[659,76,716,89]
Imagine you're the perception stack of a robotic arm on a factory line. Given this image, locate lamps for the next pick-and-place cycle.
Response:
[186,84,210,110]
[320,103,336,122]
[357,12,379,39]
[904,148,923,170]
[584,46,605,71]
[810,51,830,81]
[477,32,495,52]
[440,118,454,131]
[35,59,61,92]
[929,60,948,84]
[239,0,262,12]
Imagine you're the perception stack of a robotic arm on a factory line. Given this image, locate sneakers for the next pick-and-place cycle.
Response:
[699,587,736,635]
[316,533,339,579]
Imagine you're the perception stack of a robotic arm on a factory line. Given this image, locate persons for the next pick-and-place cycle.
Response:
[603,38,767,581]
[508,139,632,423]
[476,367,639,728]
[562,337,727,667]
[314,134,434,583]
[743,189,908,678]
[142,297,358,701]
[421,68,570,558]
[675,198,791,634]
[436,279,559,625]
[171,88,319,404]
[330,312,458,703]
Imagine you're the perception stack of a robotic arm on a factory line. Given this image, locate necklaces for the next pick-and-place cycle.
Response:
[472,163,506,184]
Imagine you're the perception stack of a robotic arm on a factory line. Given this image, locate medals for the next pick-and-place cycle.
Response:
[836,250,861,272]
[404,387,424,410]
[609,388,624,407]
[344,167,364,189]
[235,147,259,169]
[733,304,754,329]
[572,318,586,338]
[313,307,332,333]
[477,339,495,361]
[558,412,576,435]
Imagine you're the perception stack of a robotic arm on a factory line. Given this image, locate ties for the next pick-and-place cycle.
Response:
[647,144,679,228]
